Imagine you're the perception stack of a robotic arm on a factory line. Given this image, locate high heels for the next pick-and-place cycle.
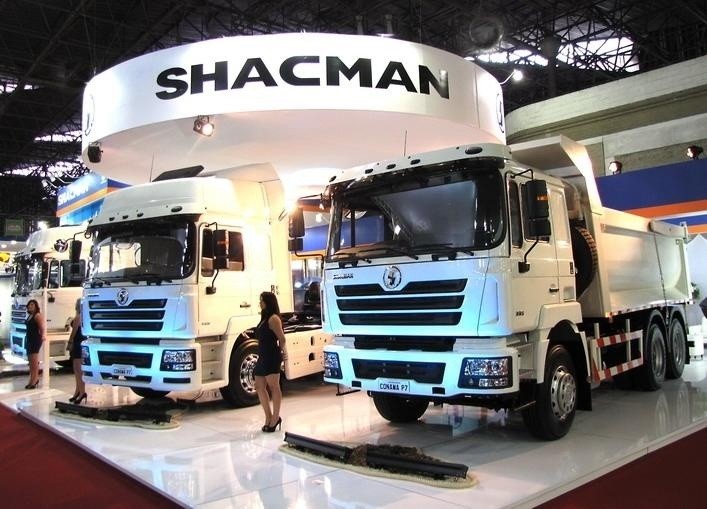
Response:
[69,392,87,405]
[25,378,40,391]
[261,416,282,433]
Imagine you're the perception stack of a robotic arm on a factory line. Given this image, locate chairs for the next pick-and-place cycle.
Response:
[689,317,707,343]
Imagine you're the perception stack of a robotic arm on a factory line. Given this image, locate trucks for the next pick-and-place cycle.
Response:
[291,140,694,441]
[73,167,328,404]
[4,223,96,372]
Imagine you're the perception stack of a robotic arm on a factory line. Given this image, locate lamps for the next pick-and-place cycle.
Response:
[500,66,523,84]
[42,167,79,189]
[193,115,214,137]
[687,145,703,159]
[88,142,103,163]
[609,161,622,174]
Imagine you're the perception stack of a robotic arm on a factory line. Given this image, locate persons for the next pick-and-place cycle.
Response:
[66,299,88,404]
[254,291,288,432]
[24,299,47,389]
